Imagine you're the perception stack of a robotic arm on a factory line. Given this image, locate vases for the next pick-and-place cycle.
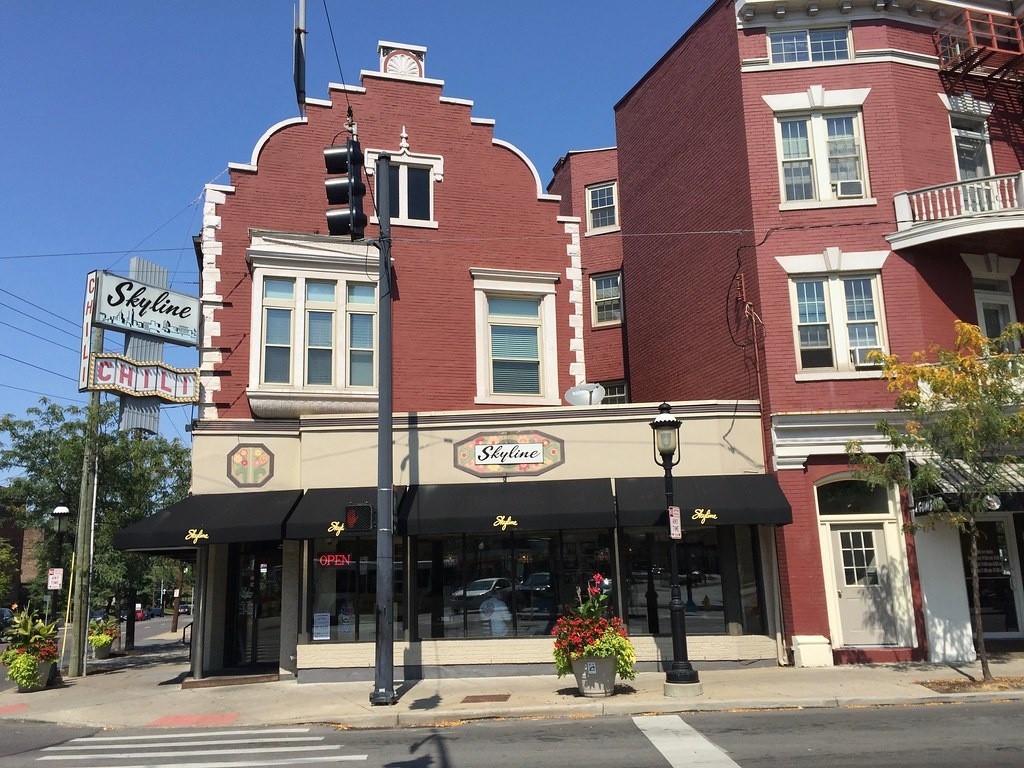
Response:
[17,660,52,692]
[569,655,616,698]
[93,642,114,659]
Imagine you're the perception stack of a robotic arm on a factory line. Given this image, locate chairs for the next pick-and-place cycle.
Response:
[463,620,493,636]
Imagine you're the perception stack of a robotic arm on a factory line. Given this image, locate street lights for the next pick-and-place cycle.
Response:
[647,400,699,684]
[47,505,71,633]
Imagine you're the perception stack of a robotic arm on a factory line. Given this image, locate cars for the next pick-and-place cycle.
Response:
[448,571,552,614]
[121,609,129,621]
[0,607,15,633]
[135,609,145,620]
[142,608,150,620]
[179,605,191,615]
[89,610,110,624]
[151,608,164,617]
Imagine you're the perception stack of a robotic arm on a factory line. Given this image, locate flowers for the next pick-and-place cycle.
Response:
[0,600,61,689]
[88,615,121,648]
[550,574,638,681]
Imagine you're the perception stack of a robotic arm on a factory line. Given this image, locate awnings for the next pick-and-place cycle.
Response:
[287,483,408,541]
[407,476,616,536]
[113,488,304,549]
[911,453,1024,519]
[615,474,794,529]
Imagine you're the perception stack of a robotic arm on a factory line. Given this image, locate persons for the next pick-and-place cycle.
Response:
[479,587,517,640]
[9,601,20,617]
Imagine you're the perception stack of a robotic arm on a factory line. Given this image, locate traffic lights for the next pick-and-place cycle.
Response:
[323,138,364,243]
